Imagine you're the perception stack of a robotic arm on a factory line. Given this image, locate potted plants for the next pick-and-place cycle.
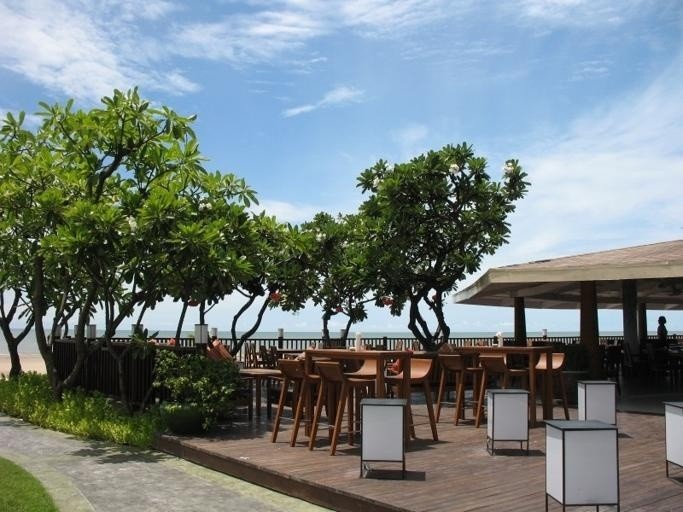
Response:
[154,349,240,435]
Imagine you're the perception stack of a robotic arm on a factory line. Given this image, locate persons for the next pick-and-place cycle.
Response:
[658,317,667,345]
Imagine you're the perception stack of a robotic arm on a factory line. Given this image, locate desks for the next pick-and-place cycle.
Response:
[544,420,621,512]
[238,368,282,420]
[664,401,683,478]
[359,398,408,478]
